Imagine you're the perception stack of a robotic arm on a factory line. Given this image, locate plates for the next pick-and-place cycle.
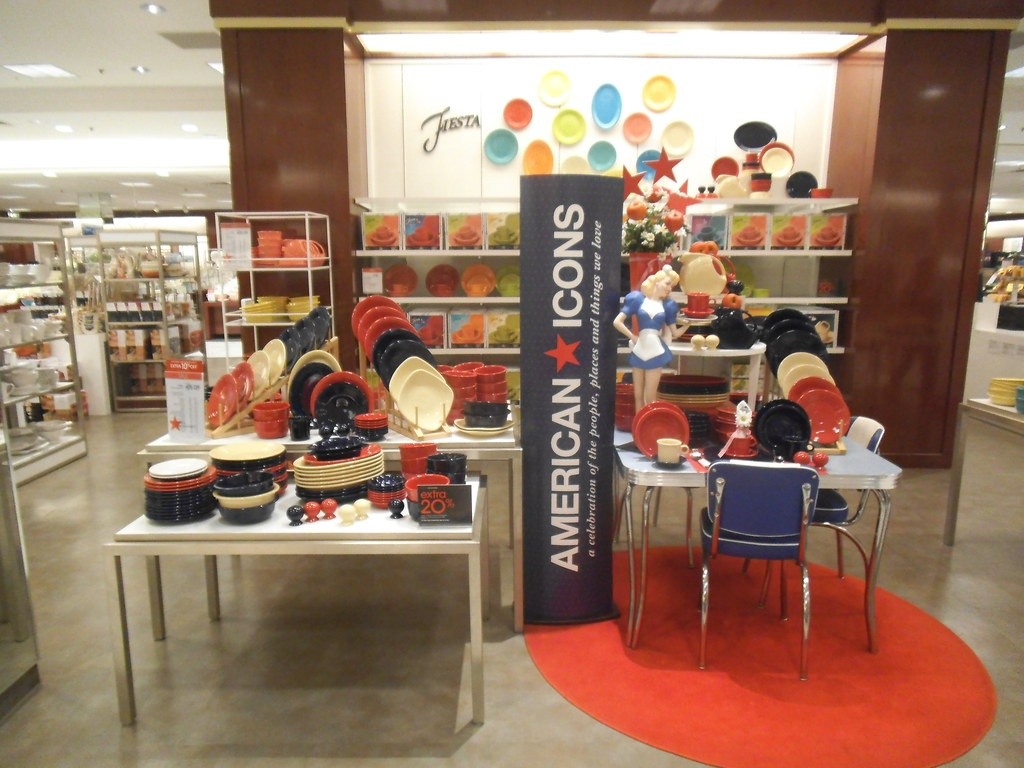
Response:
[383,263,520,297]
[695,233,722,243]
[142,441,407,526]
[654,456,687,468]
[776,234,802,245]
[815,232,840,246]
[204,294,453,431]
[736,233,763,246]
[482,70,695,179]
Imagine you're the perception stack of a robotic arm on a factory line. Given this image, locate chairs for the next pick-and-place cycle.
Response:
[738,417,886,608]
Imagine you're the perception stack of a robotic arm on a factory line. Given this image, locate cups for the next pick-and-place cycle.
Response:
[743,225,760,240]
[782,227,796,240]
[655,438,690,464]
[820,226,834,241]
[700,226,714,241]
[687,292,716,312]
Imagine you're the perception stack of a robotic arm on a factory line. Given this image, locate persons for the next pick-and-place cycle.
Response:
[614,264,691,414]
[74,263,92,305]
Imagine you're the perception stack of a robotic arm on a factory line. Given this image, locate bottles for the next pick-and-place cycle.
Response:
[695,186,706,199]
[706,186,719,199]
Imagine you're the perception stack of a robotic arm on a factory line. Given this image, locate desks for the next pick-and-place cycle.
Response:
[612,428,900,652]
[942,397,1024,548]
[106,469,490,727]
[135,417,524,634]
[698,458,818,682]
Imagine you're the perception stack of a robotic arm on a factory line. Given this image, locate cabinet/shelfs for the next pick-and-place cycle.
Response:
[0,197,855,489]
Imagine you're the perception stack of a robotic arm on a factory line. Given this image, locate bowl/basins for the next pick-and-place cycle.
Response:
[0,262,74,456]
[369,225,518,246]
[239,230,325,323]
[613,307,851,459]
[399,442,469,523]
[987,376,1024,414]
[710,120,834,199]
[436,360,511,427]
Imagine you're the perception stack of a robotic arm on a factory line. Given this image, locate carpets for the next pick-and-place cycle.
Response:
[523,547,998,768]
